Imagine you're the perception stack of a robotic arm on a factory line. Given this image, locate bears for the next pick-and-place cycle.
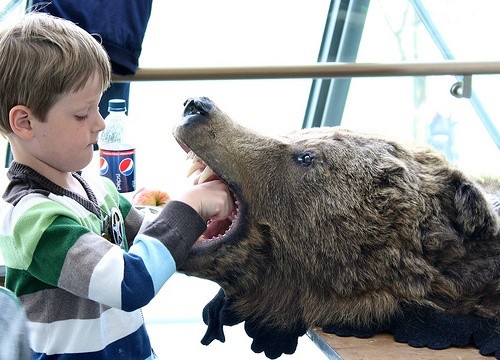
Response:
[172,97,500,360]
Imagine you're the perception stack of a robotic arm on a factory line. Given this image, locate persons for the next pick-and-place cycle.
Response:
[0,12,237,360]
[5,0,161,169]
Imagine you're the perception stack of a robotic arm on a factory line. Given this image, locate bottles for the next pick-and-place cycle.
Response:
[98,98,137,205]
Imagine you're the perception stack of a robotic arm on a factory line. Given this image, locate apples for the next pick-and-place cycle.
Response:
[133,187,171,208]
